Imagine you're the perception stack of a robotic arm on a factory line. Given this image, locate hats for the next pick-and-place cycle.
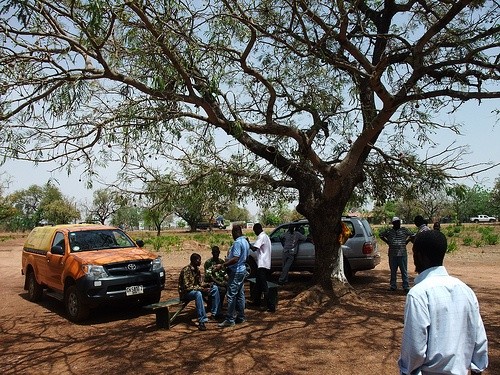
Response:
[391,217,401,223]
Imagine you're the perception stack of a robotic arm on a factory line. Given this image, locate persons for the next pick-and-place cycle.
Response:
[379,216,414,292]
[433,222,441,232]
[277,224,312,285]
[397,230,489,375]
[177,253,221,331]
[214,224,250,327]
[404,215,432,244]
[245,223,275,311]
[203,246,229,319]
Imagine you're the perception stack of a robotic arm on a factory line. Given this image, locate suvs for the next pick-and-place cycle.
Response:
[249,215,378,280]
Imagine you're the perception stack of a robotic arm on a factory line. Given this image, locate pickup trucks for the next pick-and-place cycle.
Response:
[470,214,496,224]
[22,222,166,322]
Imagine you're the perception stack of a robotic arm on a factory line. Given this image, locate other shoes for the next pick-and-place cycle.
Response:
[217,320,236,327]
[199,322,207,330]
[235,319,246,323]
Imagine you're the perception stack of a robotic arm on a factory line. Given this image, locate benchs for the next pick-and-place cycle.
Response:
[144,278,281,330]
